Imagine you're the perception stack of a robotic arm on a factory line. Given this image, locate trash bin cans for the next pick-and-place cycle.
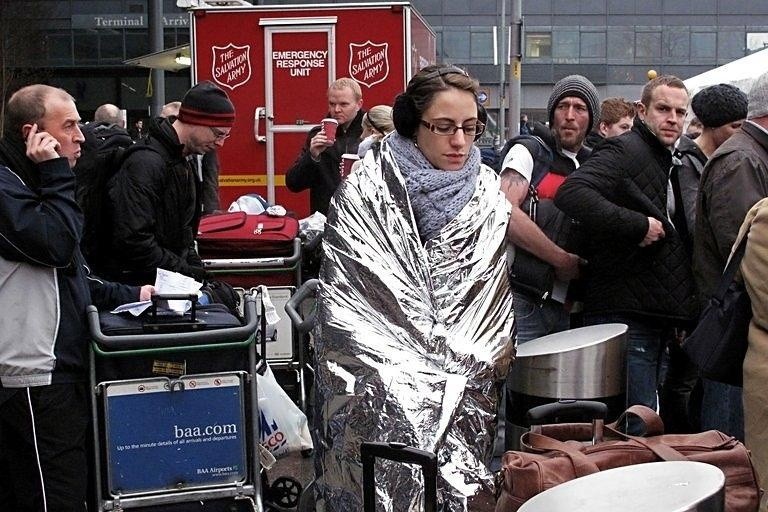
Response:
[505,323,628,452]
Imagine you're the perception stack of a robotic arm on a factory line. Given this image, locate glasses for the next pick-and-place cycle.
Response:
[419,119,486,137]
[208,126,231,141]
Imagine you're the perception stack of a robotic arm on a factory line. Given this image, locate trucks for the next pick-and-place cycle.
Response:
[187,5,438,224]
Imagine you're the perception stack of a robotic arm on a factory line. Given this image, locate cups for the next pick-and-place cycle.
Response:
[320,118,339,147]
[340,153,361,181]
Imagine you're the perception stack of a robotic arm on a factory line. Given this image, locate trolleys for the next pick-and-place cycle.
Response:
[192,235,308,416]
[85,290,301,512]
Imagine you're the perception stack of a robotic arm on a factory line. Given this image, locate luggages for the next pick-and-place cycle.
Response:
[86,291,247,382]
[197,210,299,288]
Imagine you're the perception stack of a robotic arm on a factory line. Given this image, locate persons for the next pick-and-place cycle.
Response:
[285,61,768,512]
[1,81,236,511]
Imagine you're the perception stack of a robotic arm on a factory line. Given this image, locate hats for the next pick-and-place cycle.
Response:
[692,83,748,127]
[178,81,236,128]
[547,75,602,138]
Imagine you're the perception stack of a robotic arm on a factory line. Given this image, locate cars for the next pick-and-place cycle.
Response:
[253,315,278,344]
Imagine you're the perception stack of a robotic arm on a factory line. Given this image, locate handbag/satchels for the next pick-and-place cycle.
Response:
[682,285,750,388]
[492,405,763,512]
